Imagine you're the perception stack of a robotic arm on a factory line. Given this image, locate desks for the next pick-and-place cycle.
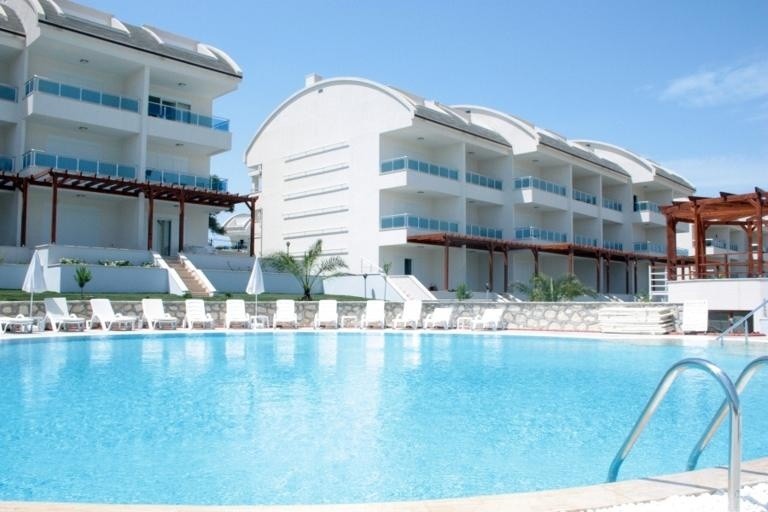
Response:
[340,315,358,329]
[249,315,270,329]
[456,317,472,330]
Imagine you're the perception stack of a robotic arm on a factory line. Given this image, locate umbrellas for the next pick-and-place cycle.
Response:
[21,248,47,316]
[245,256,267,316]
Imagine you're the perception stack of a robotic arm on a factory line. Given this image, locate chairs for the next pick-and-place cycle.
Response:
[181,298,214,332]
[1,314,35,332]
[471,308,506,332]
[360,299,386,330]
[140,297,178,332]
[88,296,138,333]
[39,297,86,333]
[313,298,339,329]
[423,307,452,329]
[223,297,251,332]
[391,299,423,329]
[271,298,298,331]
